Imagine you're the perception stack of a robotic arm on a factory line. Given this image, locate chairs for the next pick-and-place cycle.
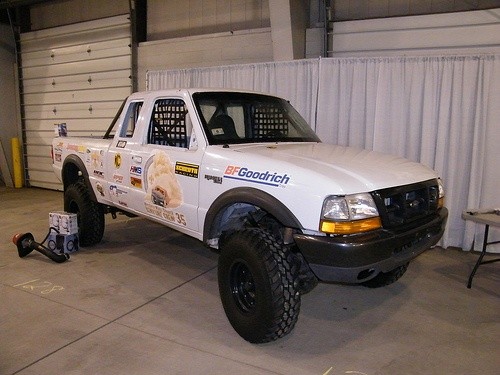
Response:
[213,114,241,138]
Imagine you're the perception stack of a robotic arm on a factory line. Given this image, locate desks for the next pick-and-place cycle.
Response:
[461,209,500,288]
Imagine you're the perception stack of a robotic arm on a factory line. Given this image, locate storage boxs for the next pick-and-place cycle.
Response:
[48,211,79,255]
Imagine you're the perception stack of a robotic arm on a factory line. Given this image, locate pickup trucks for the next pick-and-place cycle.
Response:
[50,87,449,343]
